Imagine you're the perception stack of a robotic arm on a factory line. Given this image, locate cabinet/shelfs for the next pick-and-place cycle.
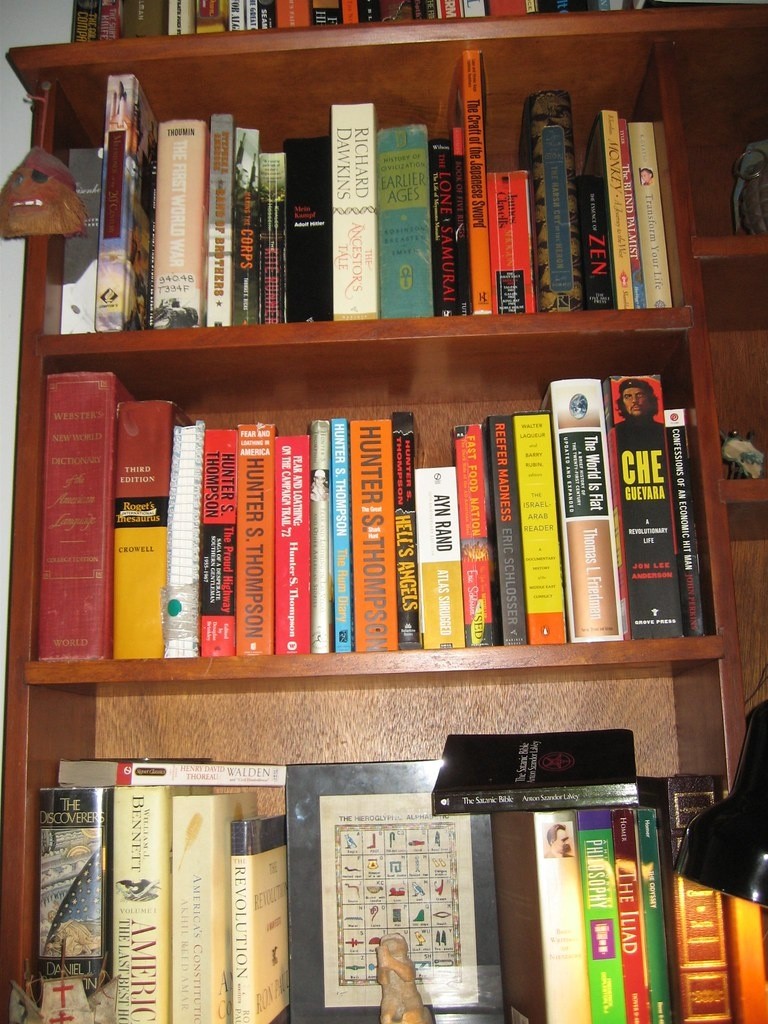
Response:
[0,0,768,1024]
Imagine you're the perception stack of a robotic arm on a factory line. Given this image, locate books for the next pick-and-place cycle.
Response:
[36,728,733,1024]
[42,0,673,335]
[37,373,705,661]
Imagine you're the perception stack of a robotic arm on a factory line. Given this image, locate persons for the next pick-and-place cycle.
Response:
[236,163,249,192]
[310,469,329,501]
[640,168,653,185]
[543,824,573,858]
[250,154,256,184]
[153,298,198,329]
[236,140,244,173]
[615,379,664,426]
